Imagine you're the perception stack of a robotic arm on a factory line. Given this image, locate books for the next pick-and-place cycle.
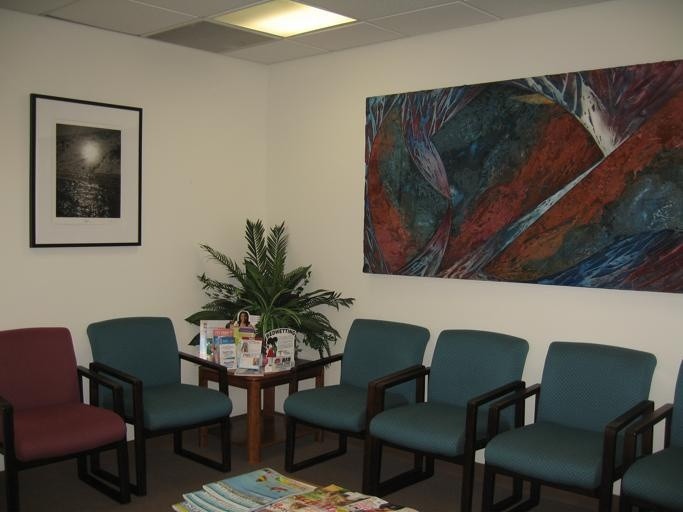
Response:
[210,326,266,377]
[169,465,421,512]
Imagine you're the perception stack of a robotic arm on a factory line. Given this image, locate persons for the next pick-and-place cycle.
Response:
[226,320,234,328]
[235,312,253,327]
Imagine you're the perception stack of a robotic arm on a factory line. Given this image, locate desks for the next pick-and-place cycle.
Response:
[199,358,324,465]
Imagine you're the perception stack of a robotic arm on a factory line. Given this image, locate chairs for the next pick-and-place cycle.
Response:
[482,342,657,512]
[1,316,131,512]
[86,317,232,496]
[619,359,683,512]
[283,319,430,495]
[371,329,529,512]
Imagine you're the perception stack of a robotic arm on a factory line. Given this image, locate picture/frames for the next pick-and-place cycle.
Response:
[29,91,142,248]
